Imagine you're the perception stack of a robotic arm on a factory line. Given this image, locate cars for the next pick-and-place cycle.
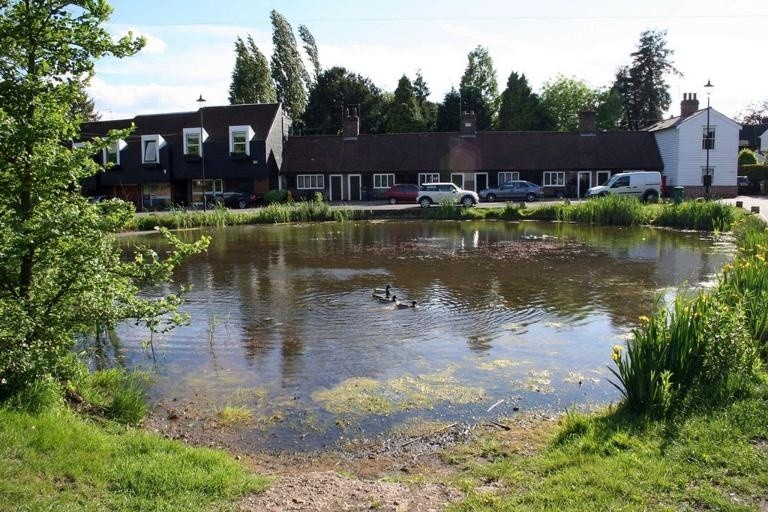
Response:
[385,184,422,204]
[479,180,544,202]
[416,183,480,207]
[201,187,265,209]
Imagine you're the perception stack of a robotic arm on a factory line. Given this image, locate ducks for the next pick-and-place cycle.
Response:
[394,300,418,309]
[371,292,392,299]
[374,284,392,293]
[379,294,397,303]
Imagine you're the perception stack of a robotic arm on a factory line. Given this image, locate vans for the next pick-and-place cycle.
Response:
[586,171,662,205]
[738,176,753,195]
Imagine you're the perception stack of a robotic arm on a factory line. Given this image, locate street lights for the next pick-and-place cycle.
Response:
[197,94,207,209]
[704,80,715,200]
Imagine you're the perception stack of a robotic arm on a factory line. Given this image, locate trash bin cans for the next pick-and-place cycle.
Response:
[673,186,684,205]
[362,186,377,201]
[556,190,564,198]
